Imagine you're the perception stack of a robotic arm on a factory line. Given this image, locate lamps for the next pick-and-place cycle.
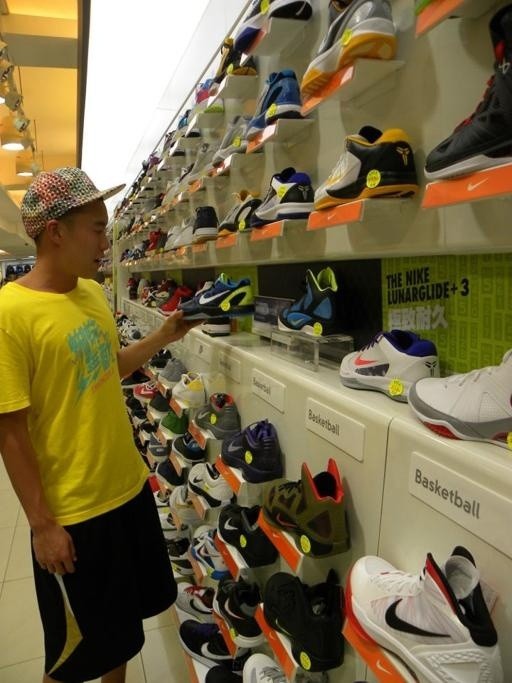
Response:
[0,34,41,178]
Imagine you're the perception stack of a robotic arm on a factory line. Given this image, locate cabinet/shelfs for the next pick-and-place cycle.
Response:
[100,0,512,683]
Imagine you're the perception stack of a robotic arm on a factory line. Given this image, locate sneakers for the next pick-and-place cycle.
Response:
[299,0,397,96]
[278,267,338,337]
[243,653,288,683]
[423,3,512,179]
[116,0,315,262]
[407,348,512,451]
[115,311,283,580]
[212,574,264,648]
[174,581,215,624]
[340,330,440,403]
[127,273,256,337]
[179,619,252,676]
[264,568,345,673]
[314,127,419,211]
[262,457,352,559]
[345,546,504,683]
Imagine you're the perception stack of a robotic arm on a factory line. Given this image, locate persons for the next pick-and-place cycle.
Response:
[0,167,202,683]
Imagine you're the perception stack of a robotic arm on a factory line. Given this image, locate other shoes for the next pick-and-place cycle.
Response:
[205,665,243,683]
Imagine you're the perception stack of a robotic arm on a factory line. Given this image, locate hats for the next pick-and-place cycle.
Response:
[21,167,126,238]
